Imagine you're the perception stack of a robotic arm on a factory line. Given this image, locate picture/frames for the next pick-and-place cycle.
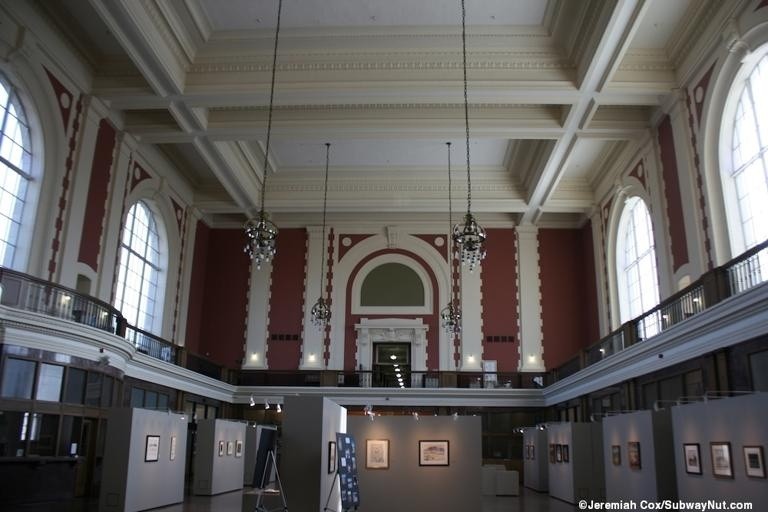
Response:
[365,439,449,469]
[218,440,243,458]
[328,441,336,474]
[525,442,766,479]
[144,435,160,463]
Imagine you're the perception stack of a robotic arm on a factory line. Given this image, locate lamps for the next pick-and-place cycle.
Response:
[242,1,489,335]
